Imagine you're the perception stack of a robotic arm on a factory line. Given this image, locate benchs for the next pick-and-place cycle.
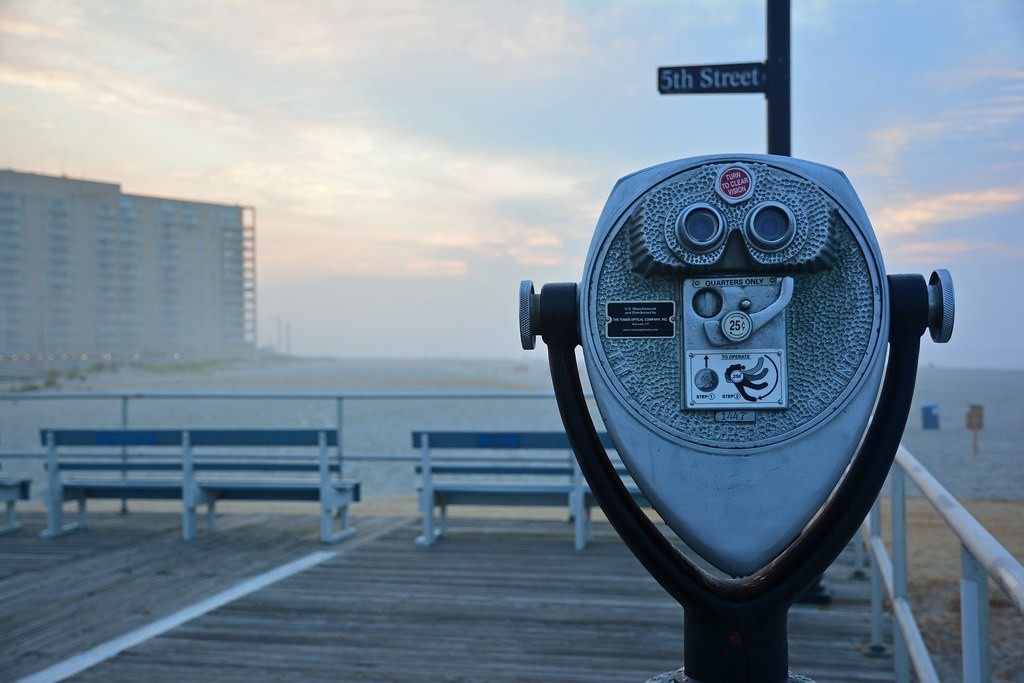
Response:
[411,429,654,552]
[39,428,361,544]
